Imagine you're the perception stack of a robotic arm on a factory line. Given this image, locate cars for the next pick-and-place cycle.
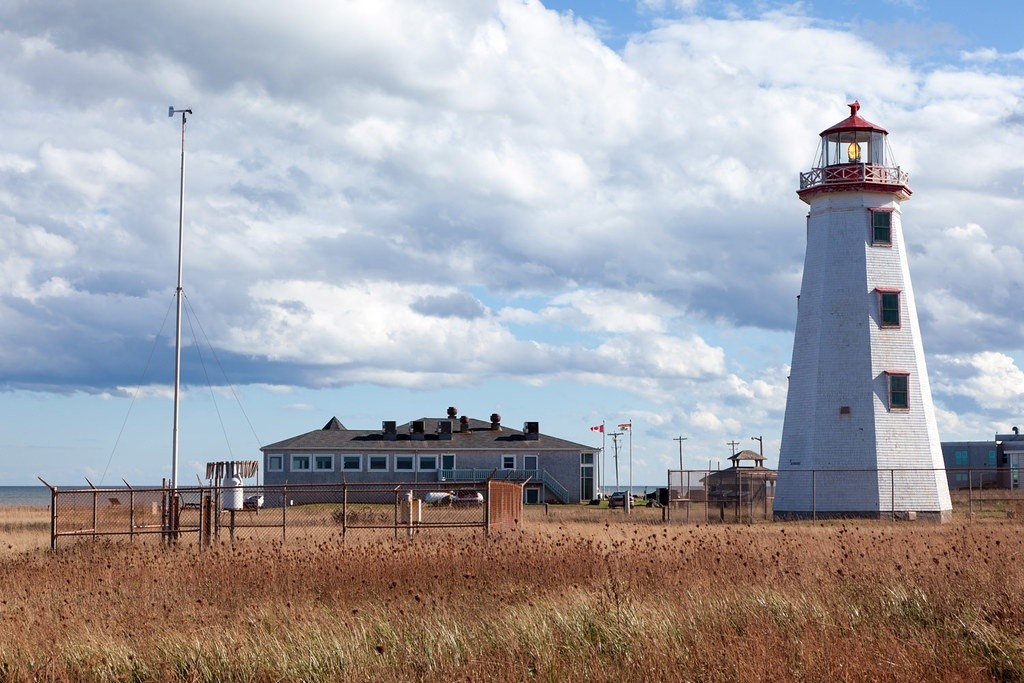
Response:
[609,491,635,509]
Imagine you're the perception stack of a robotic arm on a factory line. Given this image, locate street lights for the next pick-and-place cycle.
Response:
[750,436,763,467]
[168,106,193,498]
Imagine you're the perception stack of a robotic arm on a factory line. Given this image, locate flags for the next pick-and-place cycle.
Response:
[618,424,630,431]
[590,425,603,432]
